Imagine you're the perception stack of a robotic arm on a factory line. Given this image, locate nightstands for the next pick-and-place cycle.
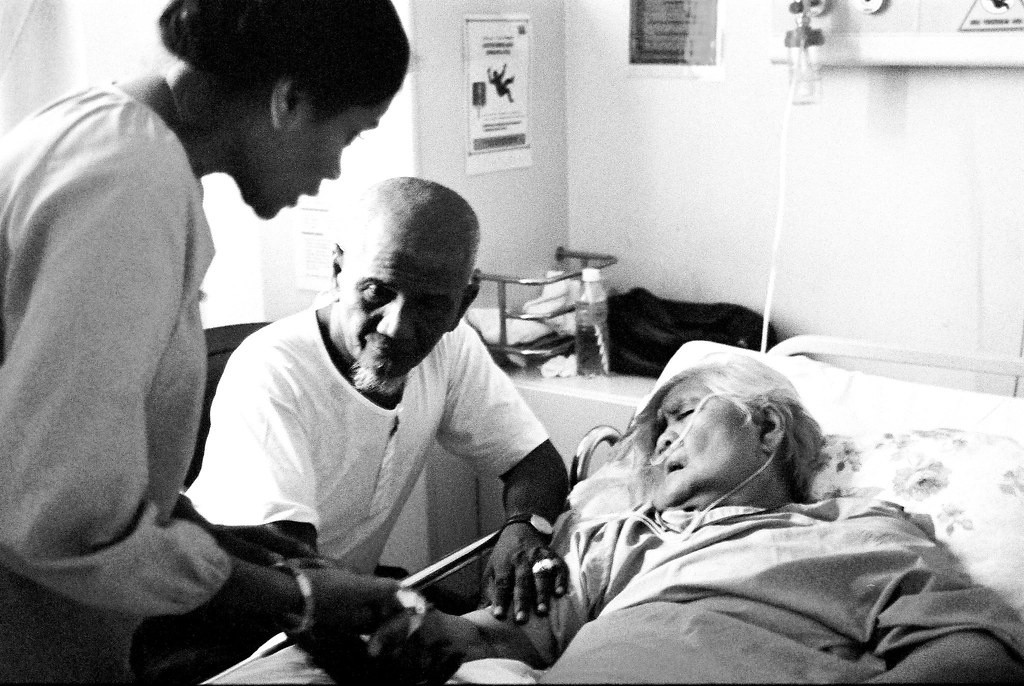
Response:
[475,359,659,603]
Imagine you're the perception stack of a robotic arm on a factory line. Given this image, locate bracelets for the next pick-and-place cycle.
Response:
[266,560,317,633]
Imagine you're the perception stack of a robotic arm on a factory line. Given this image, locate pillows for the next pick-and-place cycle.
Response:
[551,339,1024,625]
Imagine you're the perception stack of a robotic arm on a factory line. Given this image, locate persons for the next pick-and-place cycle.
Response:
[185,173,572,630]
[0,0,412,686]
[365,356,1024,685]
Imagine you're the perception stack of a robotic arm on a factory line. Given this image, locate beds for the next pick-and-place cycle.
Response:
[194,332,1024,686]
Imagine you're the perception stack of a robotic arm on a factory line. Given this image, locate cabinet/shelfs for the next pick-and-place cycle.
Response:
[464,247,619,359]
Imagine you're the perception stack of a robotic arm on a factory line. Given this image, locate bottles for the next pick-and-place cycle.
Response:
[574,267,610,378]
[786,27,825,106]
[563,279,582,335]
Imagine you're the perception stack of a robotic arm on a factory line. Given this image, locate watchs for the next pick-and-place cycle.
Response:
[501,512,554,545]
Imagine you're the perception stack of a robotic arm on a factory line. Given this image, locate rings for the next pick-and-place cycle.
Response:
[531,555,557,574]
